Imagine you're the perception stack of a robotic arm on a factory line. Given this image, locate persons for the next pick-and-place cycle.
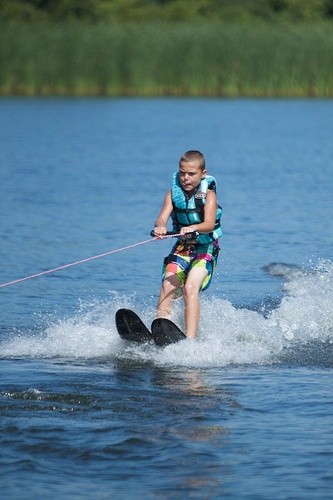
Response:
[154,150,223,339]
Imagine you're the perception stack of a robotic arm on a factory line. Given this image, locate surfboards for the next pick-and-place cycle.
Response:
[151,318,186,346]
[115,308,152,342]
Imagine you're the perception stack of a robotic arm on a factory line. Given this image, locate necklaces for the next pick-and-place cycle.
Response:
[181,182,196,190]
